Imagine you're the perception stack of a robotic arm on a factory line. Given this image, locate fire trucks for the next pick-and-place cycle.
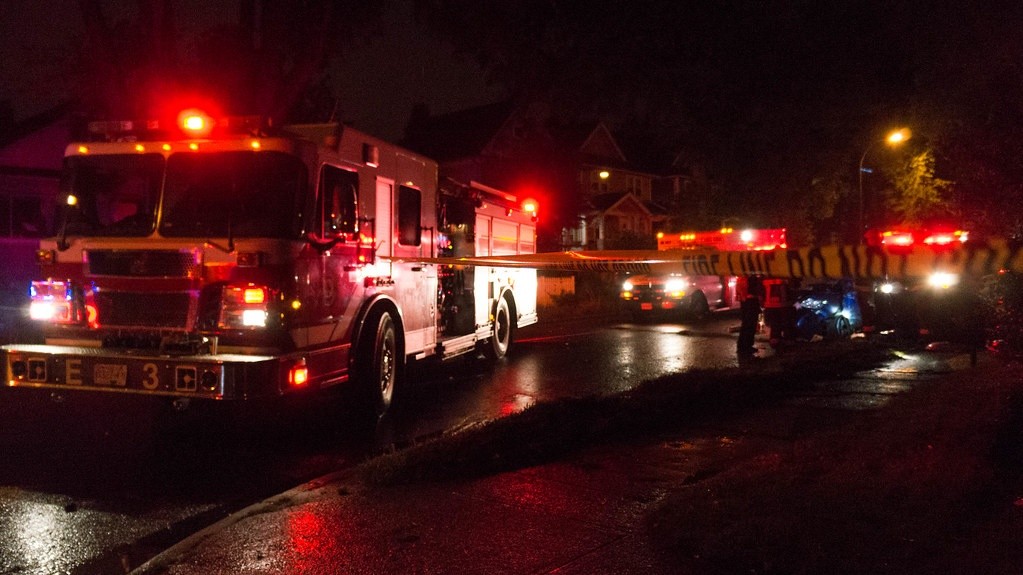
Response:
[1,108,537,424]
[621,219,990,347]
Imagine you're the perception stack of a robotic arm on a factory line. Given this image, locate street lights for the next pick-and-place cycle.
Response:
[862,127,913,243]
